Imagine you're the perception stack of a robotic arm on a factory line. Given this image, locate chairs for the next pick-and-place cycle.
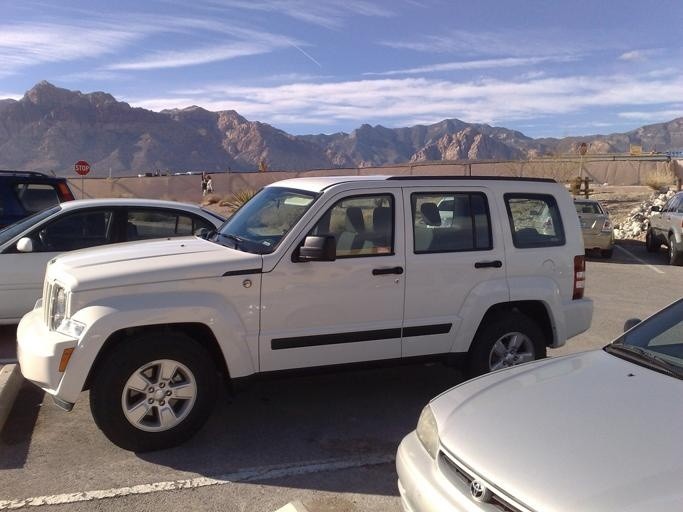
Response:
[413,202,443,250]
[335,206,392,256]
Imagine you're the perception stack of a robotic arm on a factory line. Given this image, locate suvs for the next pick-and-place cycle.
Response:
[15,175,592,454]
[0,169,74,229]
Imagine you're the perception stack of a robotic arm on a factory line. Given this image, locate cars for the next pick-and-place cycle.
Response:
[395,297,683,512]
[425,197,455,229]
[646,192,683,265]
[0,198,259,326]
[530,199,615,258]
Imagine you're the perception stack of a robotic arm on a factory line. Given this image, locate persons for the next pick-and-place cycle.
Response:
[206,174,214,195]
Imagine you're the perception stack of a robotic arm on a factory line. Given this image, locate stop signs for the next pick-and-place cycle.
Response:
[74,160,90,175]
[579,143,587,155]
